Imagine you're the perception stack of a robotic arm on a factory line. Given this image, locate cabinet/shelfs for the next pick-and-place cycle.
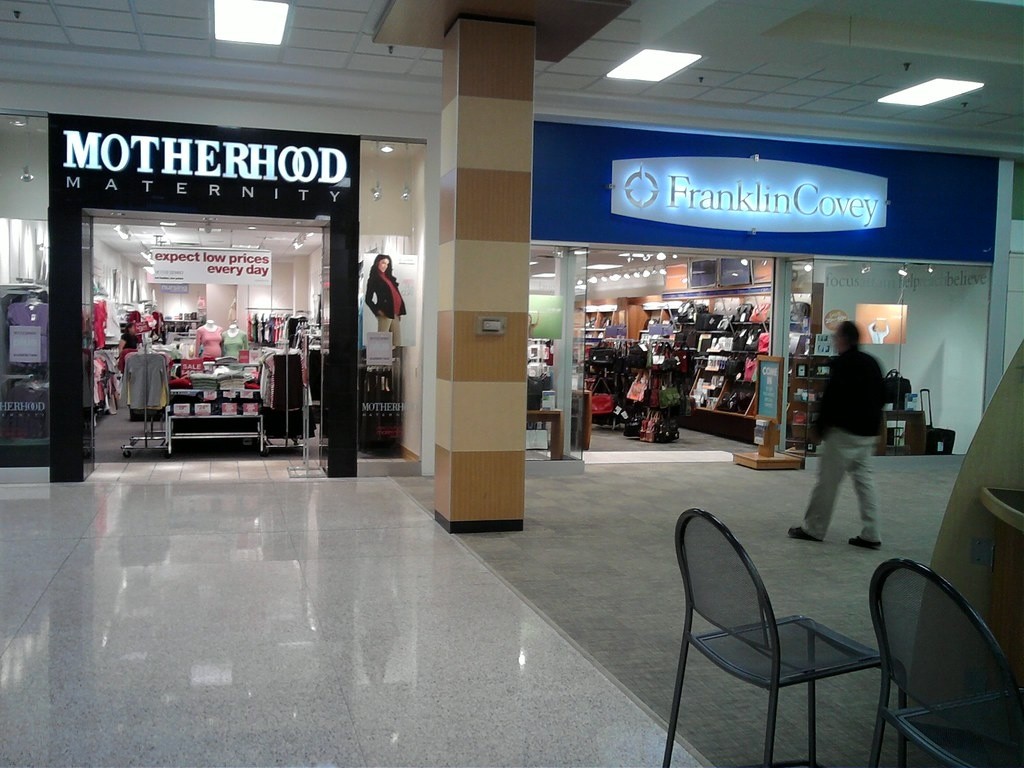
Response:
[165,319,200,338]
[586,310,626,338]
[584,337,688,444]
[638,293,770,444]
[881,411,927,456]
[786,355,839,456]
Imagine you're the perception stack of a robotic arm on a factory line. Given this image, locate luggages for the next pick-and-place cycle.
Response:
[920,388,955,456]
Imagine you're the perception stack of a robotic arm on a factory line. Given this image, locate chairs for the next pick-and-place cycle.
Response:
[661,508,908,768]
[869,558,1024,768]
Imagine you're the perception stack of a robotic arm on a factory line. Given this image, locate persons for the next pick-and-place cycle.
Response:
[195,320,248,357]
[119,323,138,353]
[788,320,887,549]
[365,254,407,346]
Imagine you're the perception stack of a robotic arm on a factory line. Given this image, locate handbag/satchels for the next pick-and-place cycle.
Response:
[526,337,554,411]
[883,370,903,401]
[573,298,811,444]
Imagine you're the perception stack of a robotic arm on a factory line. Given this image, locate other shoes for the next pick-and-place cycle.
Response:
[787,527,823,542]
[848,536,881,547]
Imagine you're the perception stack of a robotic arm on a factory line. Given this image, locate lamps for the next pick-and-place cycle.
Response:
[861,263,871,274]
[370,141,382,201]
[898,265,908,276]
[20,116,33,182]
[401,143,411,201]
